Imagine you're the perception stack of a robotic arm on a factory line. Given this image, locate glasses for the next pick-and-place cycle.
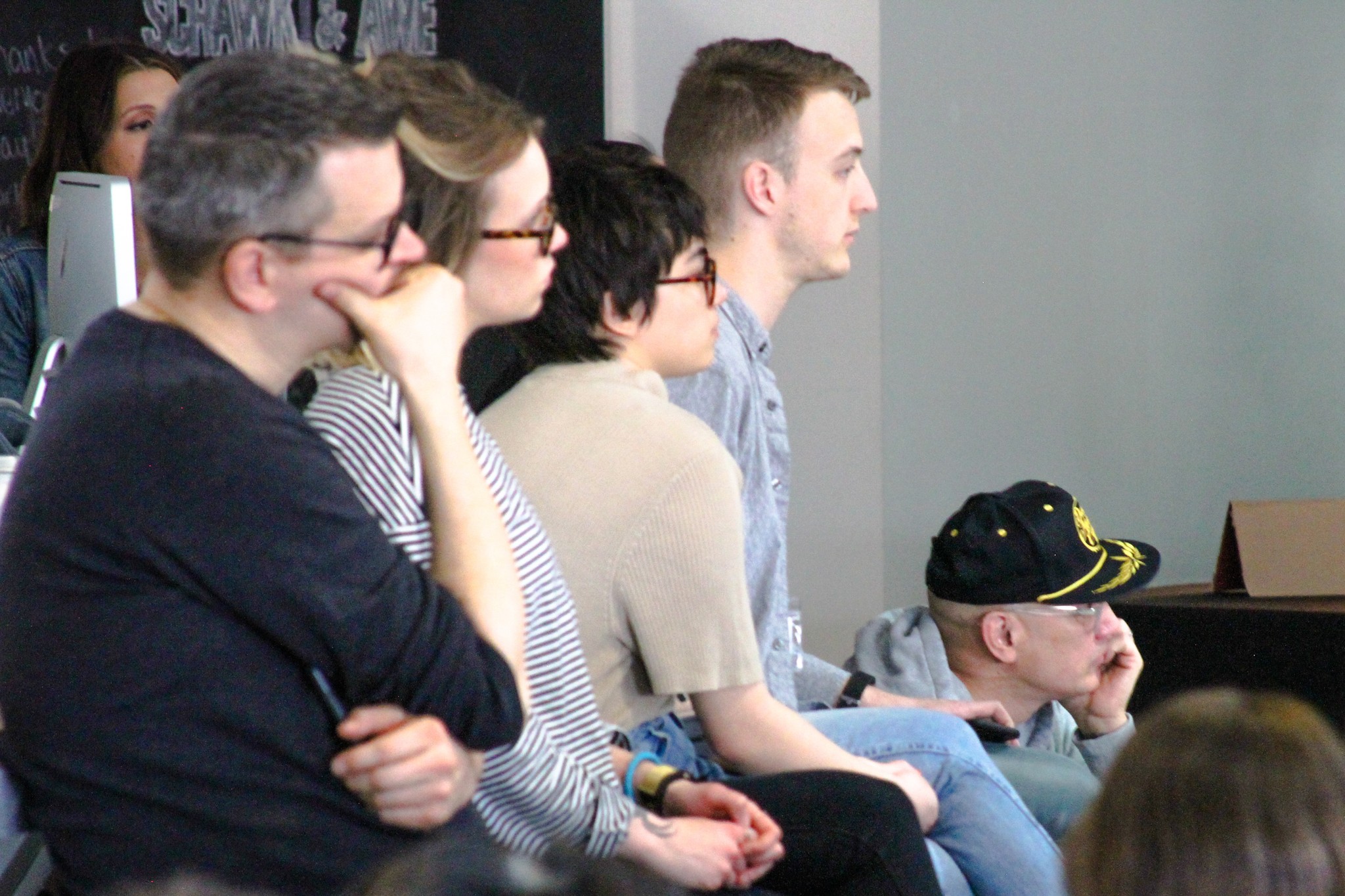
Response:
[254,208,406,268]
[1006,604,1104,636]
[653,258,715,306]
[480,202,559,255]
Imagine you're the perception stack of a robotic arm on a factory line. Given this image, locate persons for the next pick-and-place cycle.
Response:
[661,36,1106,867]
[842,480,1161,793]
[1062,685,1345,895]
[474,153,1081,896]
[2,53,546,896]
[276,52,942,895]
[0,42,189,456]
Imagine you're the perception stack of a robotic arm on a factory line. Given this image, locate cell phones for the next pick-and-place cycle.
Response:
[308,665,368,746]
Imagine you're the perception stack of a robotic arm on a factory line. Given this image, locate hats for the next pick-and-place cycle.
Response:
[924,479,1162,605]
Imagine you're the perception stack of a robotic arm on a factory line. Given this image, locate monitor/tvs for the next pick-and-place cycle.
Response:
[20,170,138,416]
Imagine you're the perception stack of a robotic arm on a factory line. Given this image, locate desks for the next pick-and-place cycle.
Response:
[1112,578,1345,730]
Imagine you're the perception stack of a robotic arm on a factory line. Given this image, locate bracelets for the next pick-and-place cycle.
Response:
[624,750,695,820]
[834,669,876,710]
